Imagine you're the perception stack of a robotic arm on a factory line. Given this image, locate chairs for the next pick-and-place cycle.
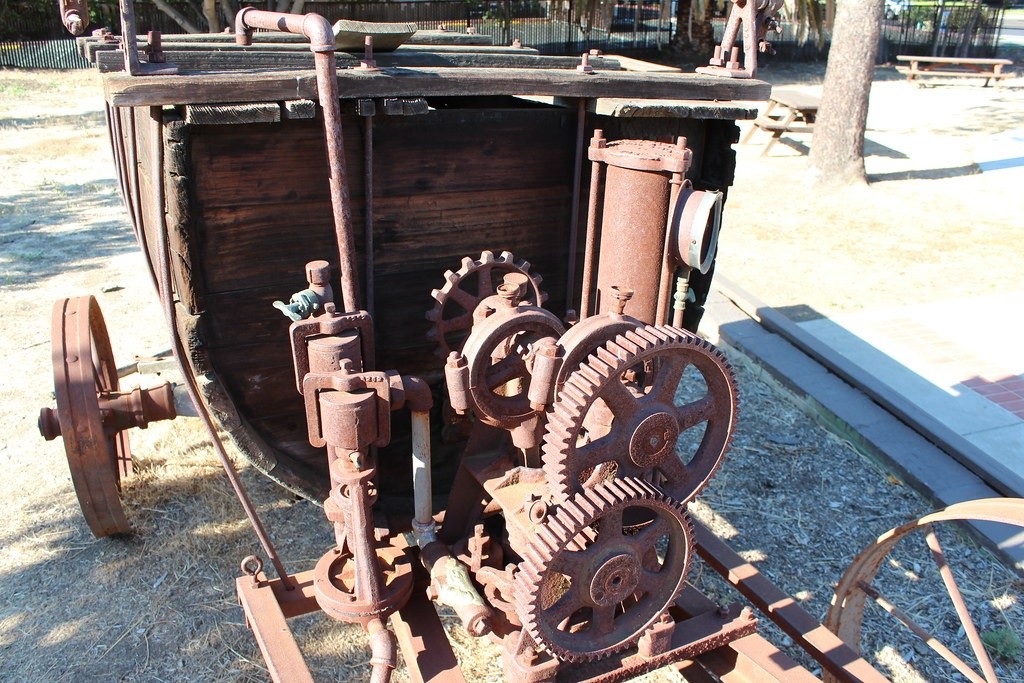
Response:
[754,116,814,132]
[894,65,1017,87]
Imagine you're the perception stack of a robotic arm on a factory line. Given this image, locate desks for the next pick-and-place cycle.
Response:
[587,54,682,72]
[742,90,822,158]
[896,55,1014,72]
